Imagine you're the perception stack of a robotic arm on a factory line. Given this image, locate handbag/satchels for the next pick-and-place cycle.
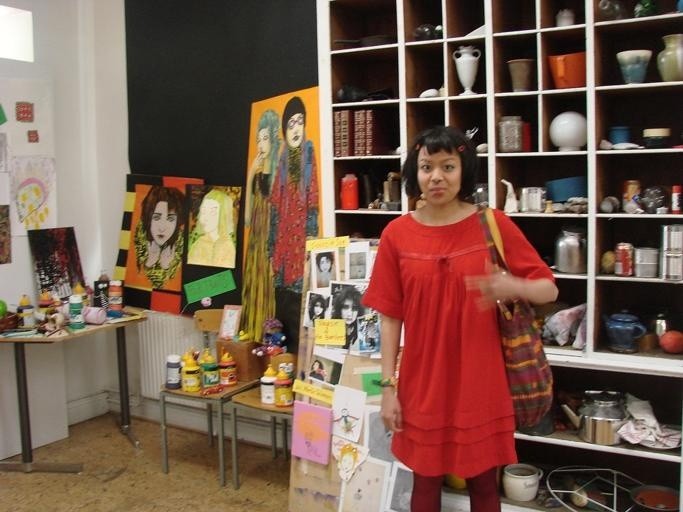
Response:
[497,298,554,434]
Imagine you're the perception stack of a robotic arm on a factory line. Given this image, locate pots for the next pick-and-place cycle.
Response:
[502,463,544,501]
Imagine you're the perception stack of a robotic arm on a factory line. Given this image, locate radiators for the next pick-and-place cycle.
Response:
[137,309,292,427]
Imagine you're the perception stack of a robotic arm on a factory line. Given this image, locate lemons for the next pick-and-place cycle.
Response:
[19,295,32,307]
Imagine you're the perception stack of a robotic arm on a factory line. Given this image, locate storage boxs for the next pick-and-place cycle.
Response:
[215,339,265,382]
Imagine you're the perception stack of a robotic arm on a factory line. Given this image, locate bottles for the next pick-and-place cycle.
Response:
[15,273,124,333]
[599,180,682,214]
[498,115,532,152]
[164,347,294,407]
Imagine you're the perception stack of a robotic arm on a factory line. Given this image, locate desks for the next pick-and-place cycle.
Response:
[0,308,148,474]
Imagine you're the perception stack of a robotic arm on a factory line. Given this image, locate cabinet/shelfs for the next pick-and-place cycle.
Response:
[315,0,683,512]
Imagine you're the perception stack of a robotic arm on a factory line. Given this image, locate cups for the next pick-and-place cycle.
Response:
[506,59,534,91]
[519,186,543,212]
[617,49,652,84]
[341,177,359,211]
[633,224,683,282]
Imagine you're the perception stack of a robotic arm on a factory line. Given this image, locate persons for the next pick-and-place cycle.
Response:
[134,186,183,289]
[361,125,559,511]
[315,253,336,287]
[240,96,313,352]
[190,189,235,267]
[331,289,364,350]
[311,295,328,324]
[312,360,326,382]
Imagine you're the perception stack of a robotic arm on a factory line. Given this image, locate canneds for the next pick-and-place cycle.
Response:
[37,300,56,324]
[274,379,295,408]
[621,178,641,212]
[200,363,220,388]
[16,304,35,331]
[217,361,239,387]
[181,366,201,393]
[259,376,277,405]
[650,313,672,350]
[614,242,634,277]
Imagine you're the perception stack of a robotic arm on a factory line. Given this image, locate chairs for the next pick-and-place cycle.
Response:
[159,309,276,486]
[231,383,295,489]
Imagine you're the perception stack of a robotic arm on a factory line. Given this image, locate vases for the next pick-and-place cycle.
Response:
[657,35,683,81]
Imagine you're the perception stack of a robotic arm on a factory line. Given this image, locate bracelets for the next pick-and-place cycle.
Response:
[378,376,396,385]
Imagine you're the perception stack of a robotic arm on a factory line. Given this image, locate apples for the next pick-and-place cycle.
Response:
[0,299,7,318]
[659,329,683,356]
[599,251,615,275]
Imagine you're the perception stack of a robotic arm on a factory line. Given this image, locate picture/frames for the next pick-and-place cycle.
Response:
[218,305,243,340]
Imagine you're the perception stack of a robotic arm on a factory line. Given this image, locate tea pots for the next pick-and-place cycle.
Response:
[601,309,646,353]
[554,227,587,273]
[560,390,624,445]
